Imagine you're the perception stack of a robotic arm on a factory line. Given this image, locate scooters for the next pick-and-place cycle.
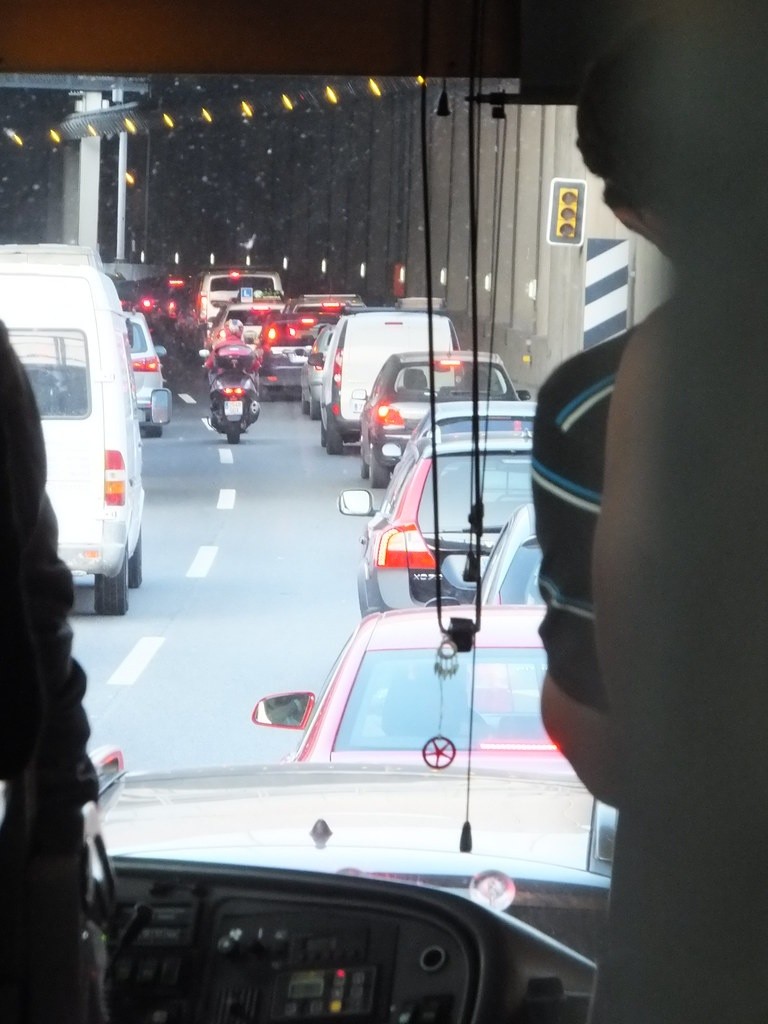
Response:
[197,341,264,444]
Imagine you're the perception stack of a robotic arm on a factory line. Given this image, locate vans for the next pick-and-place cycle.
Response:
[197,266,465,456]
[2,240,145,614]
[122,308,169,435]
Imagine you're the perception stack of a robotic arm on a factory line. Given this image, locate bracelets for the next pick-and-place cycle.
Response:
[291,699,302,711]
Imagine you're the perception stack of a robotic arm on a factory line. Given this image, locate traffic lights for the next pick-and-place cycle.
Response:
[546,177,588,249]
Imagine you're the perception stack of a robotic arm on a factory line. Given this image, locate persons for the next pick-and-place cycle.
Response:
[0,322,98,857]
[262,696,307,727]
[535,9,768,807]
[203,319,261,384]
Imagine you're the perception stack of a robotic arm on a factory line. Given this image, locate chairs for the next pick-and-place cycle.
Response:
[404,370,428,396]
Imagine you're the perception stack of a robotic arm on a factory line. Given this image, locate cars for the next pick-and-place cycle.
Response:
[422,504,546,610]
[352,346,532,489]
[379,396,537,513]
[339,431,539,618]
[248,601,589,767]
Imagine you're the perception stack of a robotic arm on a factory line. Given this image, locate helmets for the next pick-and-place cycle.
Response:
[224,319,243,337]
